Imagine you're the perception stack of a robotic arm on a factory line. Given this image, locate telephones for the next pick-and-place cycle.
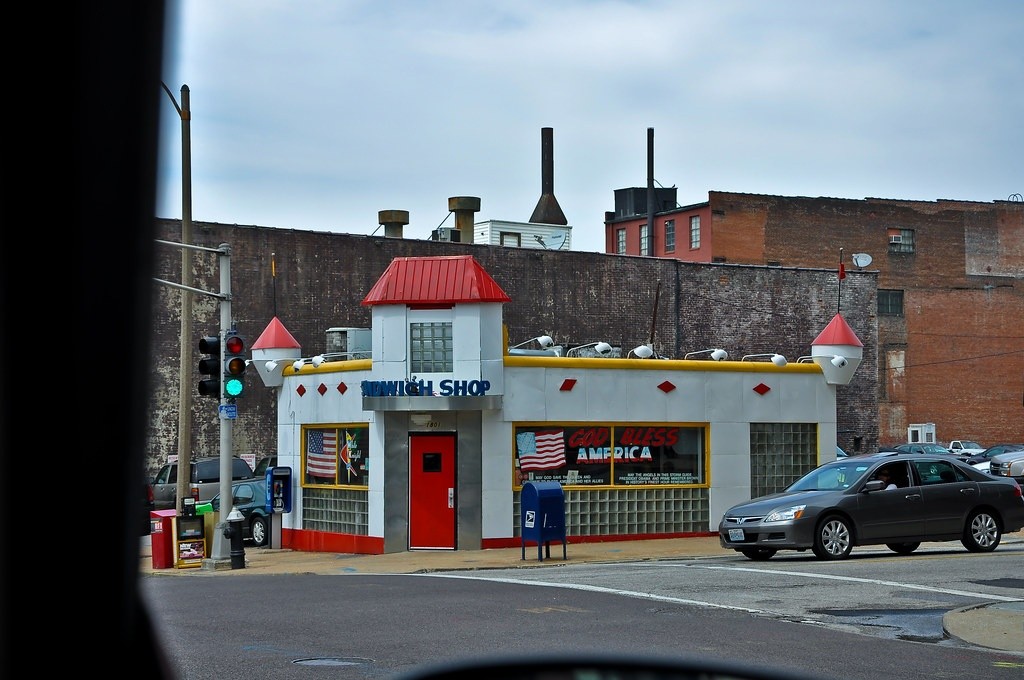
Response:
[274,480,283,497]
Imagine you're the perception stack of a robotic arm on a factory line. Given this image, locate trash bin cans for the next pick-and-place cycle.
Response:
[520,481,568,561]
[195,503,220,558]
[150,509,176,569]
[176,514,207,569]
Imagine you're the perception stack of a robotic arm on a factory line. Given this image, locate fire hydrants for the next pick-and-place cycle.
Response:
[222,505,247,570]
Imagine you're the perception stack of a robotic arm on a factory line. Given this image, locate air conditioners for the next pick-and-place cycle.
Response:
[889,235,902,243]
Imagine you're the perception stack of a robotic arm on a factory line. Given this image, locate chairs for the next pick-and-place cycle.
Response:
[935,471,955,483]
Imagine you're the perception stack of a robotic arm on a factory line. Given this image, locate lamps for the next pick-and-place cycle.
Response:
[510,336,554,350]
[245,359,312,373]
[742,354,787,366]
[567,342,613,357]
[627,345,653,359]
[684,348,728,360]
[796,355,848,368]
[312,351,372,368]
[293,354,354,372]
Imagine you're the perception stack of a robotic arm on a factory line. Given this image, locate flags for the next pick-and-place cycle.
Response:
[517,429,566,472]
[306,431,336,477]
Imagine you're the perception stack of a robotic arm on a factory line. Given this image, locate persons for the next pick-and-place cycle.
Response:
[875,468,898,491]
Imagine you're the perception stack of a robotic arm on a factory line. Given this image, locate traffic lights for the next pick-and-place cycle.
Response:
[198,336,221,401]
[222,329,247,399]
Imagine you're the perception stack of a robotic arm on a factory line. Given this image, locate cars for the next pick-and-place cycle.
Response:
[203,477,280,546]
[966,443,1024,465]
[253,453,277,483]
[717,451,1024,560]
[944,439,988,457]
[151,455,256,510]
[136,474,155,537]
[989,452,1024,476]
[894,443,970,475]
[836,446,851,469]
